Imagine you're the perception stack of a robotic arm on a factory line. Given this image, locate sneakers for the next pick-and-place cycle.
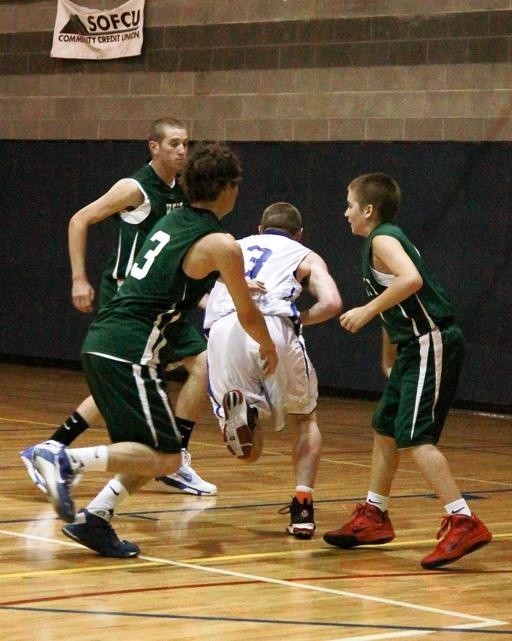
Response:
[62,508,140,557]
[421,512,493,568]
[278,490,316,539]
[154,449,217,496]
[33,444,77,523]
[223,390,255,459]
[324,501,395,546]
[19,446,49,495]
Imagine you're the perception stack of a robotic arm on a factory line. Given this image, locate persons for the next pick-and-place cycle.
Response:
[322,174,493,568]
[20,117,218,500]
[33,143,278,558]
[202,202,344,539]
